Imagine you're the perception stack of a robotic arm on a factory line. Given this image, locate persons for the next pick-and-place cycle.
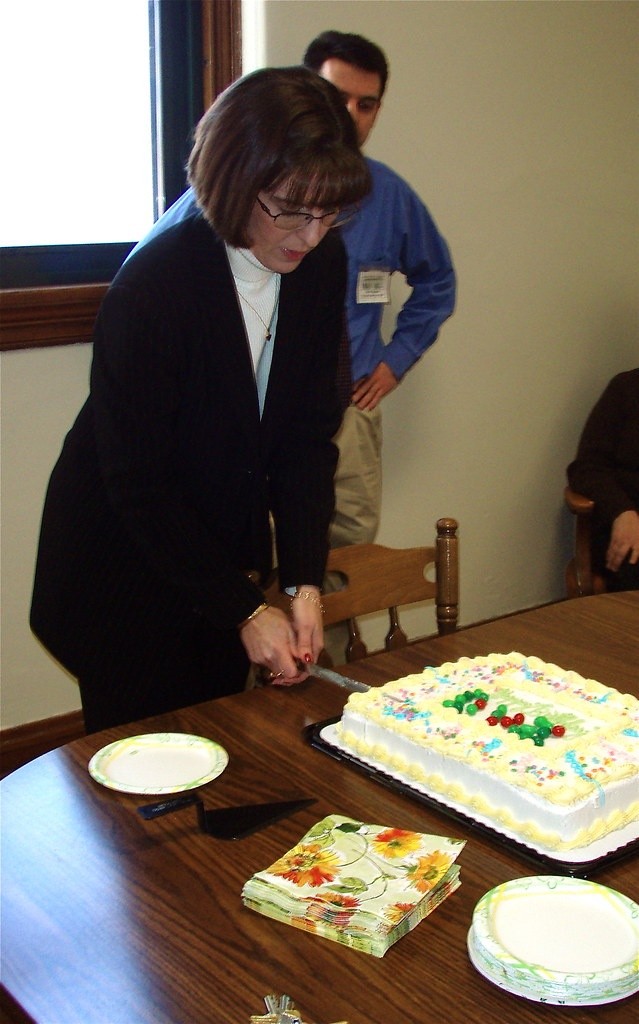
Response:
[566,367,639,596]
[29,66,356,731]
[119,30,460,668]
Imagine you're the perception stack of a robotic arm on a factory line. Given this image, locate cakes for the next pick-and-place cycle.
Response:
[337,653,638,851]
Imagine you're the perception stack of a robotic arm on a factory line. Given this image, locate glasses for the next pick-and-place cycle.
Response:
[256,195,361,231]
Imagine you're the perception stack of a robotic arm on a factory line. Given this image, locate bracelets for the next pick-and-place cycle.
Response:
[235,602,269,629]
[290,591,325,613]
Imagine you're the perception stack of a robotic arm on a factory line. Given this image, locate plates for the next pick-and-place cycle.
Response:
[465,875,639,1005]
[87,732,229,794]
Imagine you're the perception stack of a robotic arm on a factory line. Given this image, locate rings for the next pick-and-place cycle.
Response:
[269,669,285,678]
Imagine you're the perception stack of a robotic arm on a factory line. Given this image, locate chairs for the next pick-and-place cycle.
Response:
[265,512,460,676]
[564,487,608,598]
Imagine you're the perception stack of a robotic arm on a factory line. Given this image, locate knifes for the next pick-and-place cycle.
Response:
[292,658,371,694]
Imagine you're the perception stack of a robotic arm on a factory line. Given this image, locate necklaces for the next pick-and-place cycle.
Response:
[233,273,279,341]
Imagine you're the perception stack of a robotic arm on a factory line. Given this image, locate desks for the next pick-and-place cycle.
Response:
[0,579,637,1022]
